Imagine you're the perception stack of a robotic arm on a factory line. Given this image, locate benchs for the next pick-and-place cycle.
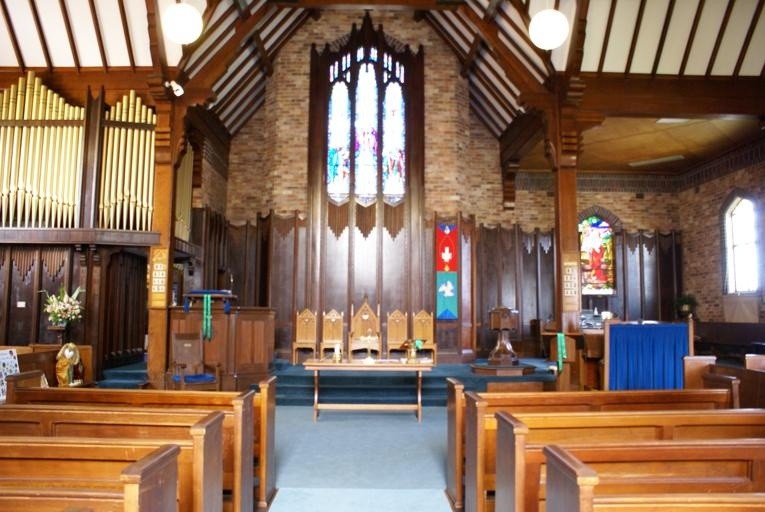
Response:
[444,355,765,512]
[0,344,277,512]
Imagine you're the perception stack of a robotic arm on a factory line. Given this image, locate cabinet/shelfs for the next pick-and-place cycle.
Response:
[168,305,279,373]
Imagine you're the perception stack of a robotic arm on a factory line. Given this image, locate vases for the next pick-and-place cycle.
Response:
[52,318,68,327]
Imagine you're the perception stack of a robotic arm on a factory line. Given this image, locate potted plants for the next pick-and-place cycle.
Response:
[672,293,701,313]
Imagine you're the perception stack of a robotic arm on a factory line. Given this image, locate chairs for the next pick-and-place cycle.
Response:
[292,294,437,365]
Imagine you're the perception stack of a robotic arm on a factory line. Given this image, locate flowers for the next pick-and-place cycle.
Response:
[35,286,86,327]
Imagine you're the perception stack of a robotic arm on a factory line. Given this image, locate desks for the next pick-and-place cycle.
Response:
[304,365,432,422]
